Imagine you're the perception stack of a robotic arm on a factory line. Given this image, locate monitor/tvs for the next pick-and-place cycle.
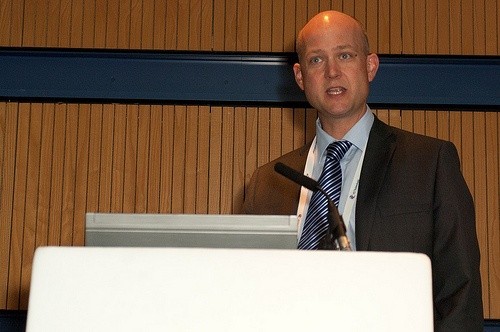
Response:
[85,213,299,250]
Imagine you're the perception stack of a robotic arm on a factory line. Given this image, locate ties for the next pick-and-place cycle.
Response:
[296,139,353,250]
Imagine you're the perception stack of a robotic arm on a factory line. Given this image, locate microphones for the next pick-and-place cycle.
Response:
[274,162,351,251]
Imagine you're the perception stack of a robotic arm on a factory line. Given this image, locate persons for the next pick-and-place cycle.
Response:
[240,9,483,332]
